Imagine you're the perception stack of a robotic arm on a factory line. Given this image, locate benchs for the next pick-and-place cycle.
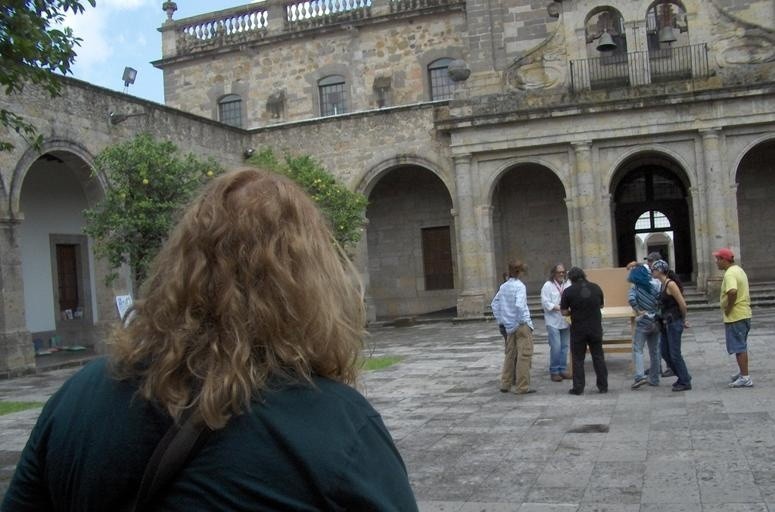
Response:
[569,267,637,379]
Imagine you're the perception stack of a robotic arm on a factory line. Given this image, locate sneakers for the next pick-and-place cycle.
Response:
[732,371,741,381]
[728,375,752,387]
[633,366,692,391]
[527,385,537,392]
[551,374,562,381]
[561,372,573,379]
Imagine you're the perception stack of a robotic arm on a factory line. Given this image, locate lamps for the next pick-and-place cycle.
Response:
[121,66,138,95]
[243,147,256,159]
[109,105,162,125]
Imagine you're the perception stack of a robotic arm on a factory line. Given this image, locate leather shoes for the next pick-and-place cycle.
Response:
[570,389,583,394]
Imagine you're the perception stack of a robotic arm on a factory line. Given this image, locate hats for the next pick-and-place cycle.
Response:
[712,248,734,262]
[644,252,663,261]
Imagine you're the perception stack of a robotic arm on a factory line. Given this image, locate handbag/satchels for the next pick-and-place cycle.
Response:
[636,314,656,333]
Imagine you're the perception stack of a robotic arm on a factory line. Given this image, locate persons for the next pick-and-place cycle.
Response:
[712,248,753,388]
[490,251,691,395]
[0,167,420,511]
